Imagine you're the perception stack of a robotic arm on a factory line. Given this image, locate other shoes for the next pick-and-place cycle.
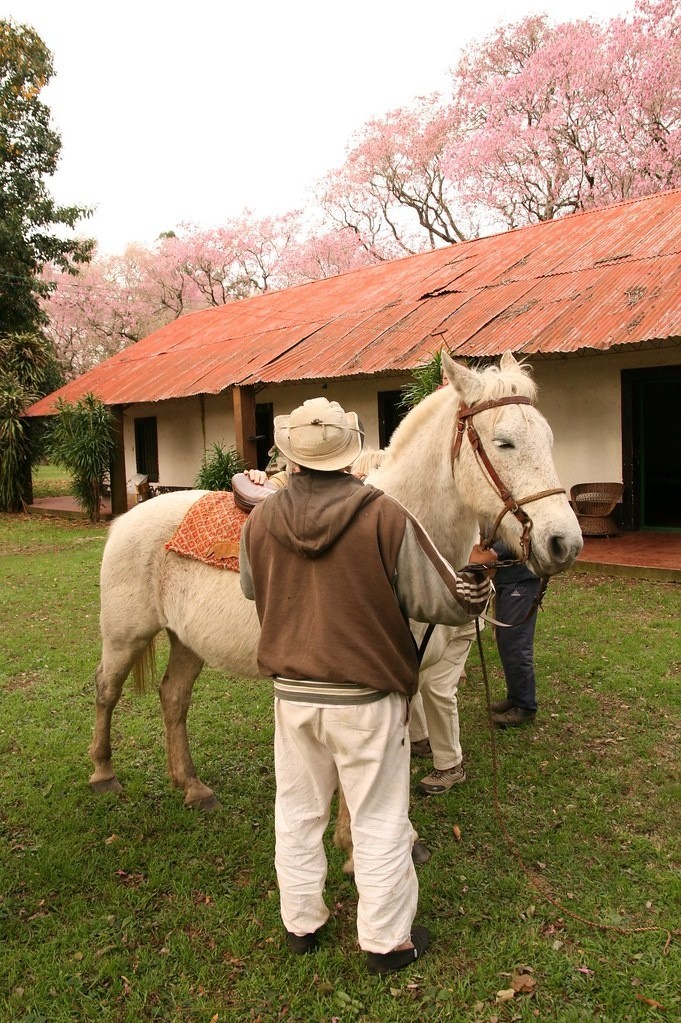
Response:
[492,707,536,726]
[488,698,513,712]
[367,926,430,978]
[286,932,314,953]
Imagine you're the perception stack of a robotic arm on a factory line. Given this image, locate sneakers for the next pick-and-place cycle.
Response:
[417,761,467,795]
[411,738,434,759]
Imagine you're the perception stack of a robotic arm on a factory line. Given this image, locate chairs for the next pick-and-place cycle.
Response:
[570,482,624,539]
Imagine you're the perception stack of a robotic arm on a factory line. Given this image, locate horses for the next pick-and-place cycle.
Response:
[91,347,584,809]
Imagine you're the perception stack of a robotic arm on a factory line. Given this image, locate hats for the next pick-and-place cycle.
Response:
[271,396,363,472]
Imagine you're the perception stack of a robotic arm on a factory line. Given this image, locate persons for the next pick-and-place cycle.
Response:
[490,521,549,726]
[238,398,498,976]
[243,469,287,489]
[411,617,485,794]
